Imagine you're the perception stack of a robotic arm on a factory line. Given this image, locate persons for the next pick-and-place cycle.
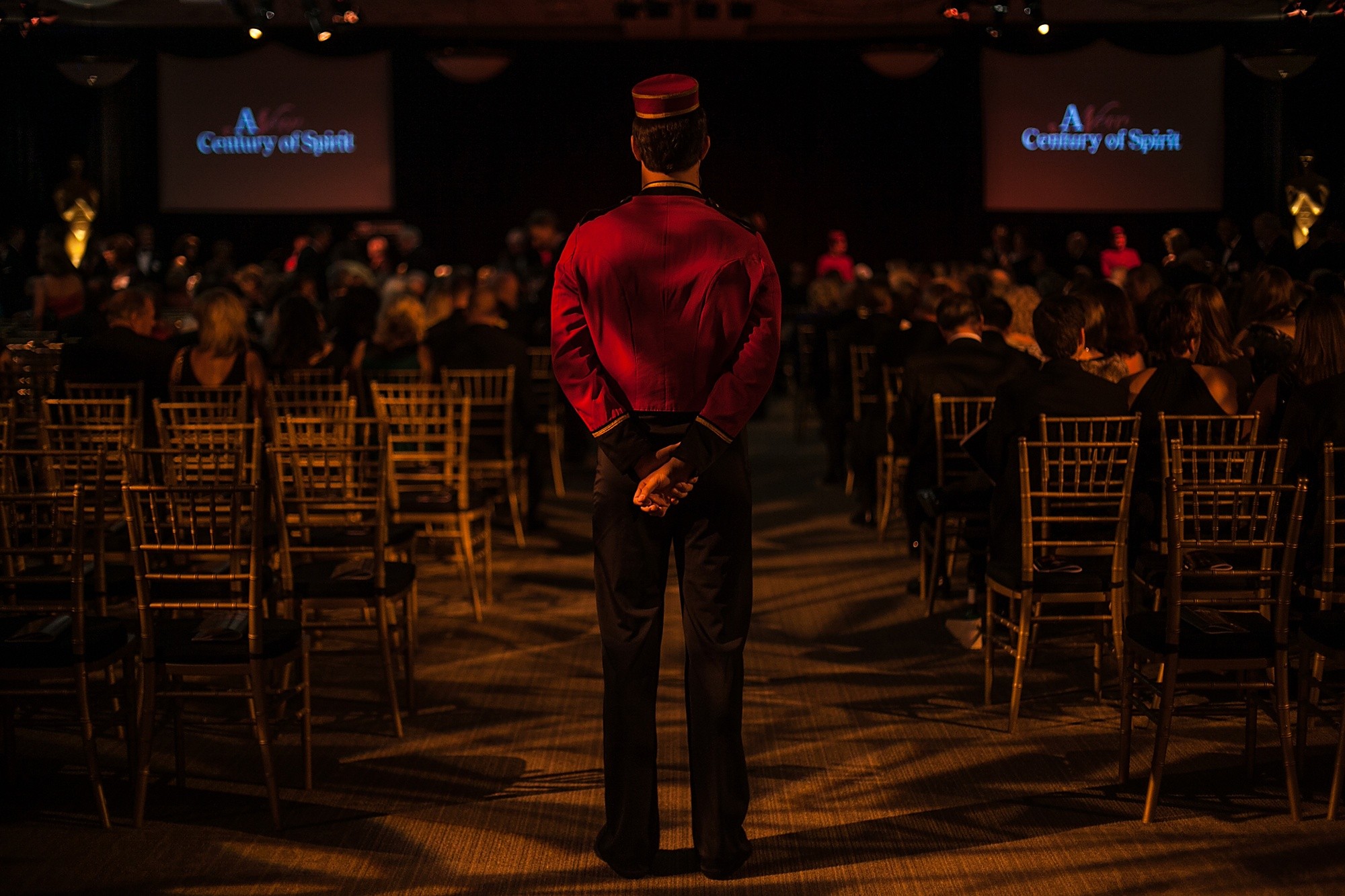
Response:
[0,220,581,523]
[549,74,781,880]
[752,214,1345,639]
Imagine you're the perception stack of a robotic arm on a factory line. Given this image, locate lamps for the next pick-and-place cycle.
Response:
[992,0,1009,14]
[333,8,345,25]
[258,9,274,20]
[306,12,331,42]
[343,9,363,25]
[248,11,270,40]
[1024,1,1037,16]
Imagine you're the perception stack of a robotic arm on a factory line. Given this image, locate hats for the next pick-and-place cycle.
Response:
[631,74,699,119]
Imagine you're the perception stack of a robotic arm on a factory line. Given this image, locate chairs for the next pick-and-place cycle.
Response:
[770,267,1345,826]
[0,319,570,842]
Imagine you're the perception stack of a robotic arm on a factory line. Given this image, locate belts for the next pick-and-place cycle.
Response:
[648,424,690,435]
